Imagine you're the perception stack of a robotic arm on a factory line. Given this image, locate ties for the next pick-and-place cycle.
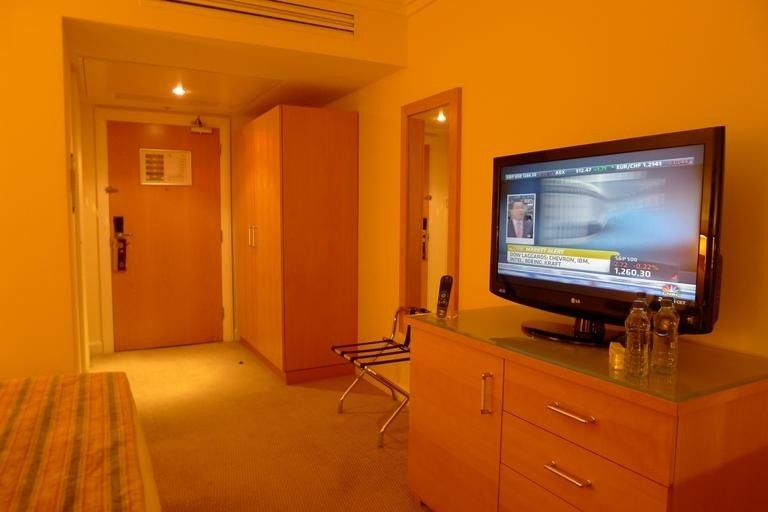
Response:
[515,223,522,238]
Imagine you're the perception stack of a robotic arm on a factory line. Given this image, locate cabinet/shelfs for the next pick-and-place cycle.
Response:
[232,102,361,388]
[399,305,768,512]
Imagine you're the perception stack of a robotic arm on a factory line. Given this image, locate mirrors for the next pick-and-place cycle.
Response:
[395,85,464,313]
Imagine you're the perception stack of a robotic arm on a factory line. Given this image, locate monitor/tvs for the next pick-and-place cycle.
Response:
[488,126,725,348]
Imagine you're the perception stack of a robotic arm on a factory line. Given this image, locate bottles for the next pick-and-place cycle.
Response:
[625,291,678,383]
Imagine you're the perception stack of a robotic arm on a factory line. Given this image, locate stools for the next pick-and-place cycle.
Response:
[331,304,432,448]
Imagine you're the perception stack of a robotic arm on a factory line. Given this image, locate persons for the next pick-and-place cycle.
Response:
[508,199,533,238]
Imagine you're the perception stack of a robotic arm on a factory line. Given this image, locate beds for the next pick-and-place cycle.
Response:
[0,371,163,511]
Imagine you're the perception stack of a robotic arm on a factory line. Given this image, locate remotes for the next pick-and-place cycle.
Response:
[436,276,452,318]
